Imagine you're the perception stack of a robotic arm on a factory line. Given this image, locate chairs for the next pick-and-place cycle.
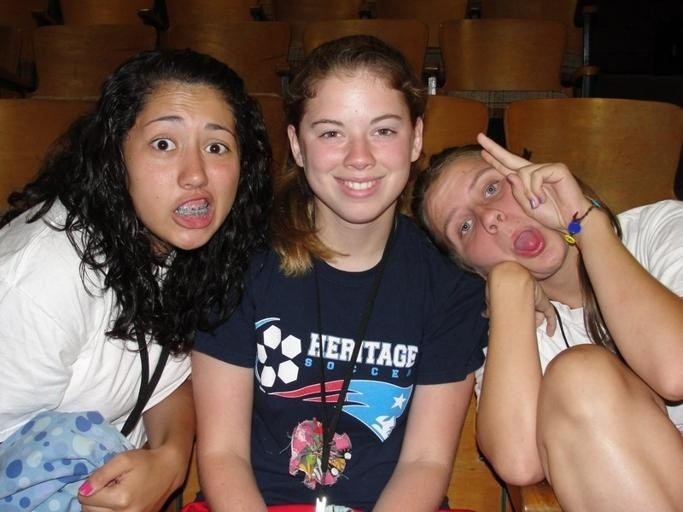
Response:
[293,4,600,117]
[444,400,563,512]
[2,101,93,219]
[163,3,290,92]
[252,94,292,186]
[170,442,202,509]
[1,2,157,100]
[407,98,683,207]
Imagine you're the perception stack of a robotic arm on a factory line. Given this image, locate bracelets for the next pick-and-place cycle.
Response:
[563,198,600,245]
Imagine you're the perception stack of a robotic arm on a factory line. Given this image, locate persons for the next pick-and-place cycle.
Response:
[190,35,489,511]
[0,25,37,98]
[0,48,274,512]
[411,133,683,511]
